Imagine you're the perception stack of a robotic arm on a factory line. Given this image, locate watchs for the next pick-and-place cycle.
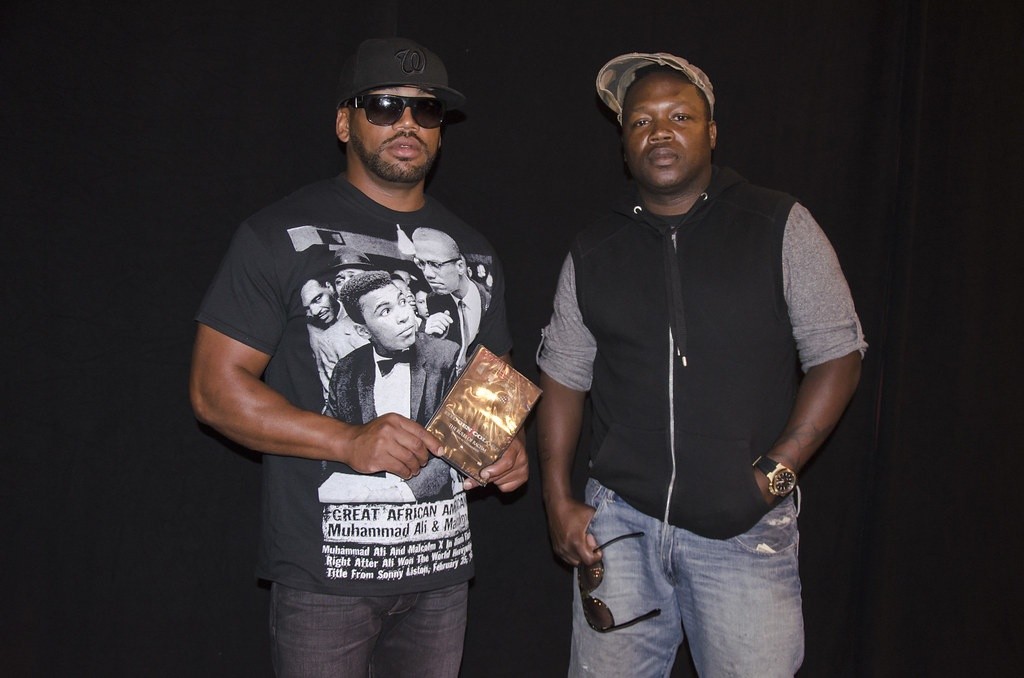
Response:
[753,455,798,498]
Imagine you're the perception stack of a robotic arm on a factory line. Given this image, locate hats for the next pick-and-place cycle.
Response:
[595,52,715,126]
[319,253,380,275]
[338,37,467,106]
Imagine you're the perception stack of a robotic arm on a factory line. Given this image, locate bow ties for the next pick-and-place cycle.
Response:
[377,350,417,377]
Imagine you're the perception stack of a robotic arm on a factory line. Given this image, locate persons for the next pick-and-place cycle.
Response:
[532,51,871,677]
[186,37,531,676]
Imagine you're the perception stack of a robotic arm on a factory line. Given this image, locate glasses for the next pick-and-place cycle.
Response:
[348,94,445,128]
[578,532,662,634]
[413,256,462,271]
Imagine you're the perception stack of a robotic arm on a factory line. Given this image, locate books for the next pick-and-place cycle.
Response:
[421,345,543,485]
